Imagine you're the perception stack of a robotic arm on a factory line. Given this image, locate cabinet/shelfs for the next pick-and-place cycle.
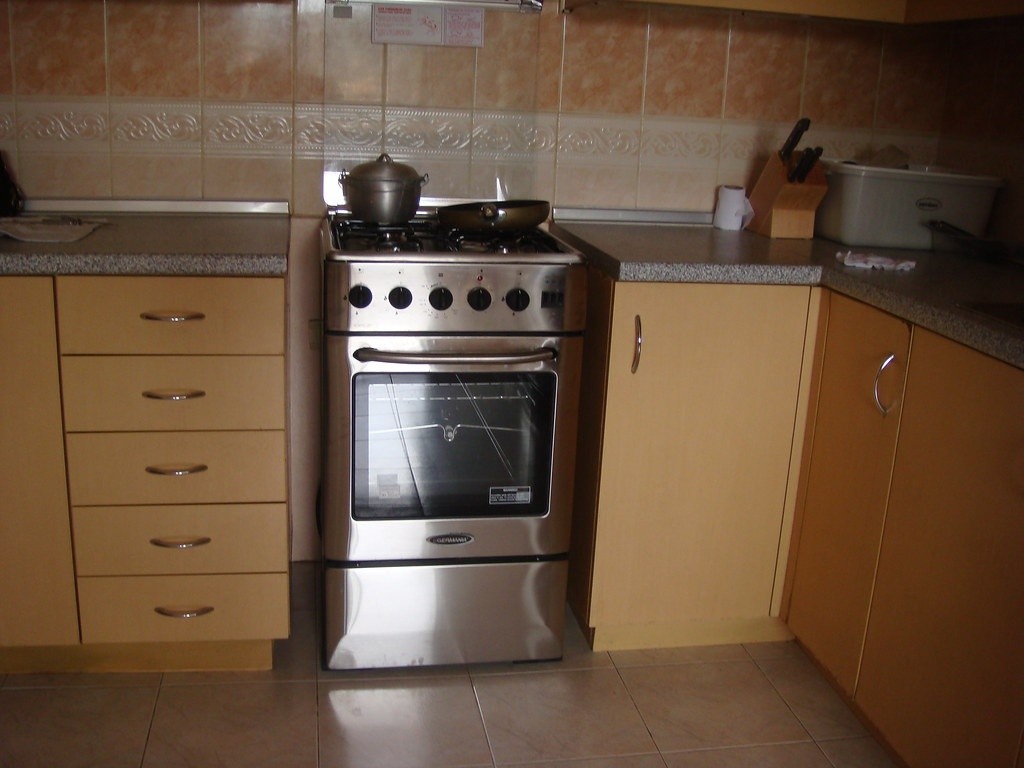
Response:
[0,275,290,674]
[555,262,1024,768]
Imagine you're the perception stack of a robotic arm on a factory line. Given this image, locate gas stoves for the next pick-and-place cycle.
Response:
[328,211,586,265]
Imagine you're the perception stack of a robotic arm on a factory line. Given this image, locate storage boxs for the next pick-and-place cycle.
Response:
[818,158,1005,250]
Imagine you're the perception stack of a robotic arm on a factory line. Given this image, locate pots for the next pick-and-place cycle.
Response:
[437,199,550,234]
[337,153,430,226]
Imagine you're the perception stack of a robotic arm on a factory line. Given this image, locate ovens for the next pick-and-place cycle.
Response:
[322,262,592,564]
